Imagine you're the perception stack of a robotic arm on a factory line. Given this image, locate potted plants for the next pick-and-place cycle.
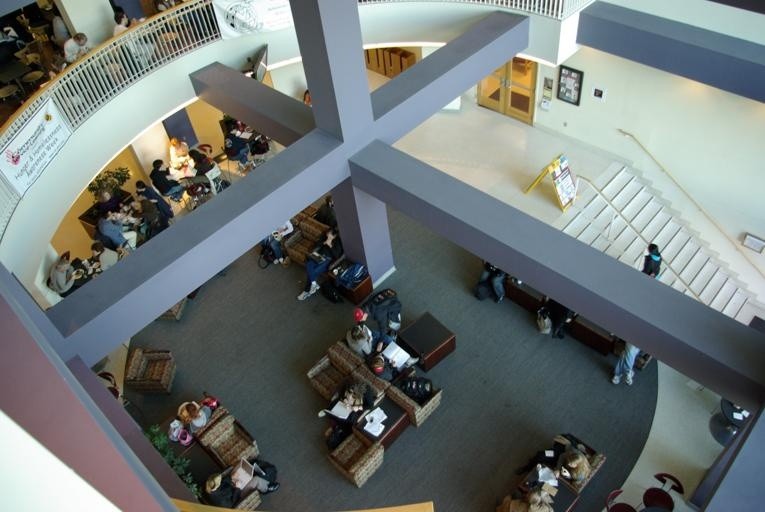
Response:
[88,167,133,203]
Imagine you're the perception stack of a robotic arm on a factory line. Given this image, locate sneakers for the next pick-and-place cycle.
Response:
[495,296,506,304]
[263,482,280,494]
[273,257,290,268]
[317,408,333,437]
[610,370,635,386]
[403,356,419,369]
[514,465,532,475]
[297,283,320,302]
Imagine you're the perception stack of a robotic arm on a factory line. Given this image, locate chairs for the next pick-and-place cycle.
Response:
[284,206,372,303]
[125,347,262,511]
[334,339,386,367]
[359,346,416,391]
[325,423,385,489]
[307,345,358,401]
[126,114,270,252]
[387,368,442,428]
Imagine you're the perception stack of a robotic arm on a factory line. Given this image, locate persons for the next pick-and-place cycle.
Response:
[367,353,419,380]
[554,451,590,485]
[497,484,555,511]
[551,300,578,340]
[353,294,401,354]
[149,159,185,202]
[0,22,20,63]
[346,326,394,359]
[186,404,214,434]
[323,376,375,449]
[188,149,215,194]
[168,137,189,164]
[642,244,661,281]
[206,472,279,509]
[112,10,155,66]
[315,196,338,226]
[50,180,174,297]
[297,228,342,301]
[261,220,294,266]
[476,258,507,304]
[611,341,640,386]
[45,11,71,49]
[64,32,97,63]
[225,124,252,170]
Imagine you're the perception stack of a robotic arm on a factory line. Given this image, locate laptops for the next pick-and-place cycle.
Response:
[304,250,326,265]
[235,458,254,490]
[324,401,353,420]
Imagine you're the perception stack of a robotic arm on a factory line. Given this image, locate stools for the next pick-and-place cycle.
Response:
[0,0,198,102]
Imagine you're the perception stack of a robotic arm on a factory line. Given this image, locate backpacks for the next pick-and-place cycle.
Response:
[361,288,401,320]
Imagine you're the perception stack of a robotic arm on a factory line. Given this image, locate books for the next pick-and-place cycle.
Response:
[381,341,411,367]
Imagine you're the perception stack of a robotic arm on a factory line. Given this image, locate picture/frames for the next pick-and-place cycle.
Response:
[741,232,765,253]
[592,86,608,103]
[557,65,584,106]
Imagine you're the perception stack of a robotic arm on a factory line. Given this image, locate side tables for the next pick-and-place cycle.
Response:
[356,396,410,452]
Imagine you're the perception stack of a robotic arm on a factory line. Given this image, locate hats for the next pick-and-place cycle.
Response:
[352,307,364,322]
[373,367,384,373]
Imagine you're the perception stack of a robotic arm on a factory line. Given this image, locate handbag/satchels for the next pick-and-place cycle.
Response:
[177,429,194,447]
[248,458,278,482]
[322,281,341,303]
[327,423,352,451]
[401,377,433,397]
[537,308,552,335]
[473,279,494,301]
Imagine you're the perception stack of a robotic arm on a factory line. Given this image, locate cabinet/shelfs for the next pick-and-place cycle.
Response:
[364,47,416,79]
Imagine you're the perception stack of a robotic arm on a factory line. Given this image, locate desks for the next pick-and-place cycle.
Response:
[78,190,135,240]
[504,429,608,512]
